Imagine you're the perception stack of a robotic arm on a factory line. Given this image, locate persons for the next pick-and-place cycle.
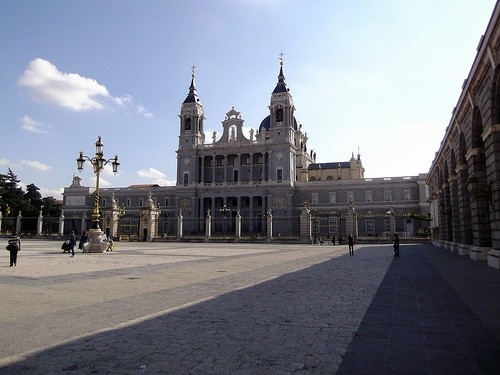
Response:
[8,231,21,267]
[61,230,88,257]
[104,233,114,252]
[392,234,399,257]
[347,233,354,256]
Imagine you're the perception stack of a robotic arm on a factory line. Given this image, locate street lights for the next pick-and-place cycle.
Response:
[76,136,121,230]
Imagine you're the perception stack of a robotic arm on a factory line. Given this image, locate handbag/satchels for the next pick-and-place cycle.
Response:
[6,245,10,251]
